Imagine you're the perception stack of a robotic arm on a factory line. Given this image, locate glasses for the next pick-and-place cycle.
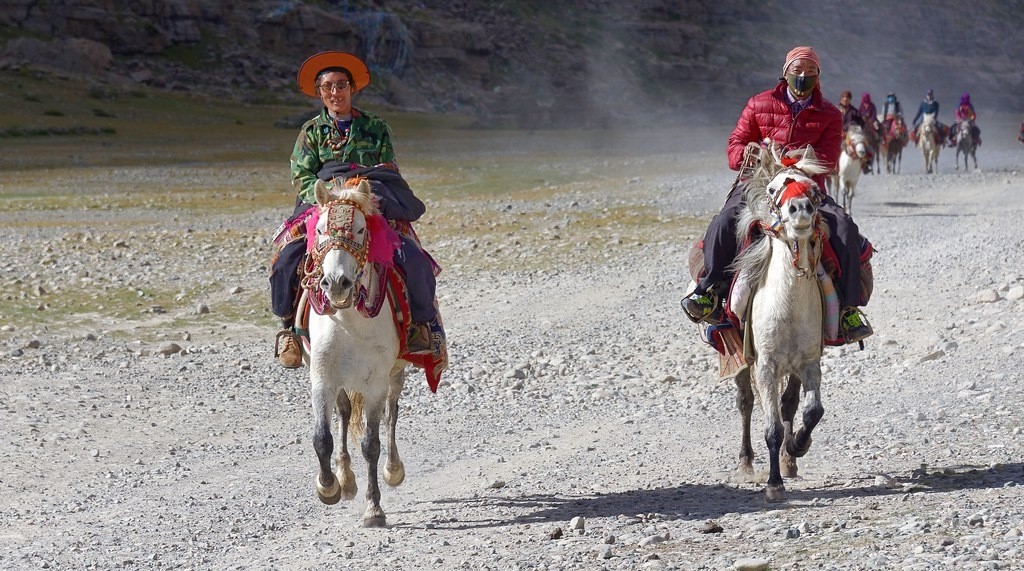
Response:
[318,80,350,90]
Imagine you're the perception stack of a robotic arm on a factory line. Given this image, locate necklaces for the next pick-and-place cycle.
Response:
[326,120,352,149]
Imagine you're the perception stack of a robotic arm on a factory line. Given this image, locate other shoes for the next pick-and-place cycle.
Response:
[863,168,871,174]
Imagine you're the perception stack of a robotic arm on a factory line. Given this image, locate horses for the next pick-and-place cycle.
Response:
[826,112,977,221]
[309,179,408,530]
[730,144,825,503]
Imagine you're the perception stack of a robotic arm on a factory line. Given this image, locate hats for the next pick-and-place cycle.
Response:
[783,46,822,78]
[927,90,933,95]
[863,94,869,99]
[887,92,895,97]
[297,51,370,98]
[840,92,851,99]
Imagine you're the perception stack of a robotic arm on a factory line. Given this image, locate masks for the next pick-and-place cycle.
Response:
[784,71,818,97]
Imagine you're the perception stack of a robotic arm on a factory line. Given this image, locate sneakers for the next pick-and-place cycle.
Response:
[279,328,302,368]
[409,321,431,349]
[843,310,870,338]
[687,290,714,319]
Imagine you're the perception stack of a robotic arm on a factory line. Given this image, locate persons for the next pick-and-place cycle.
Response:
[273,50,436,369]
[836,89,982,175]
[681,45,873,346]
